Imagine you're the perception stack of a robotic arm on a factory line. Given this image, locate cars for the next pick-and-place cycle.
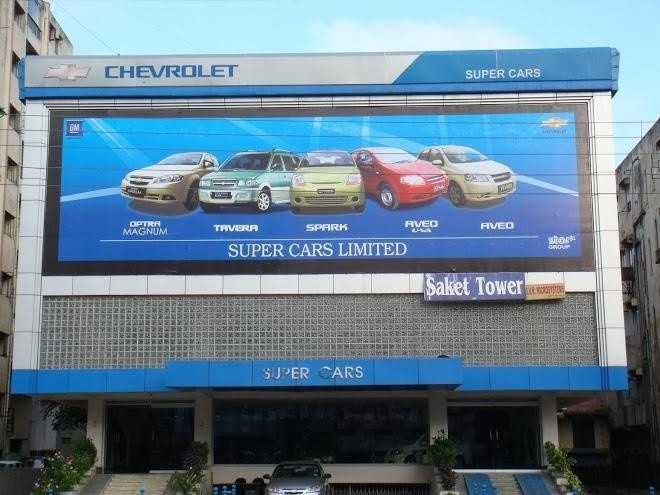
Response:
[263,461,332,495]
[198,149,300,212]
[351,147,449,210]
[291,150,365,212]
[417,145,516,207]
[120,153,219,211]
